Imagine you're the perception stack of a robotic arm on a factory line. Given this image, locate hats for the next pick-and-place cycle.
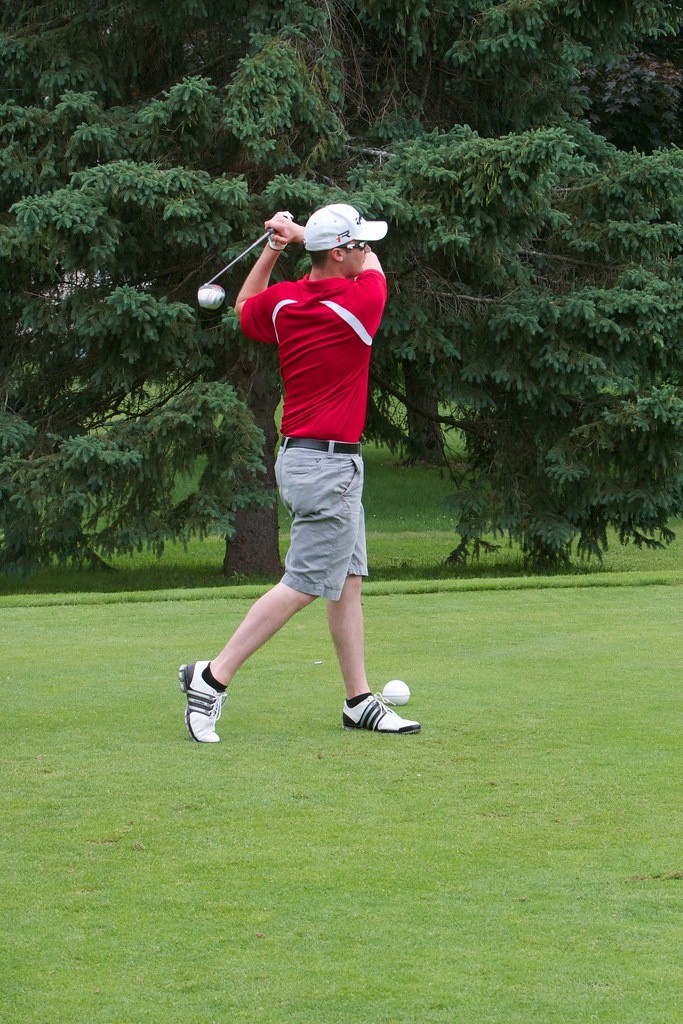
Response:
[303,203,388,251]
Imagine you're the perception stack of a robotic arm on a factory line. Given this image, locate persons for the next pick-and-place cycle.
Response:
[177,203,420,744]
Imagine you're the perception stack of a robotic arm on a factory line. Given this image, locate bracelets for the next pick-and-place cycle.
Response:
[267,234,286,251]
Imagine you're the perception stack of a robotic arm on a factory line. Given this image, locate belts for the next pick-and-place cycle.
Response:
[280,436,361,454]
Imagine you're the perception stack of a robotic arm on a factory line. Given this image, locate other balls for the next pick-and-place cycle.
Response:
[381,679,411,706]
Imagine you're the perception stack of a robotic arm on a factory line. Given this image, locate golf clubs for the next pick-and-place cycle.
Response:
[197,212,294,309]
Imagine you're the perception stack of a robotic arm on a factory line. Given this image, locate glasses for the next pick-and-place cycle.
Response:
[337,241,367,252]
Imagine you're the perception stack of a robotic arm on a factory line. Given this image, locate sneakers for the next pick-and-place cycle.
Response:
[343,691,421,734]
[178,660,227,743]
